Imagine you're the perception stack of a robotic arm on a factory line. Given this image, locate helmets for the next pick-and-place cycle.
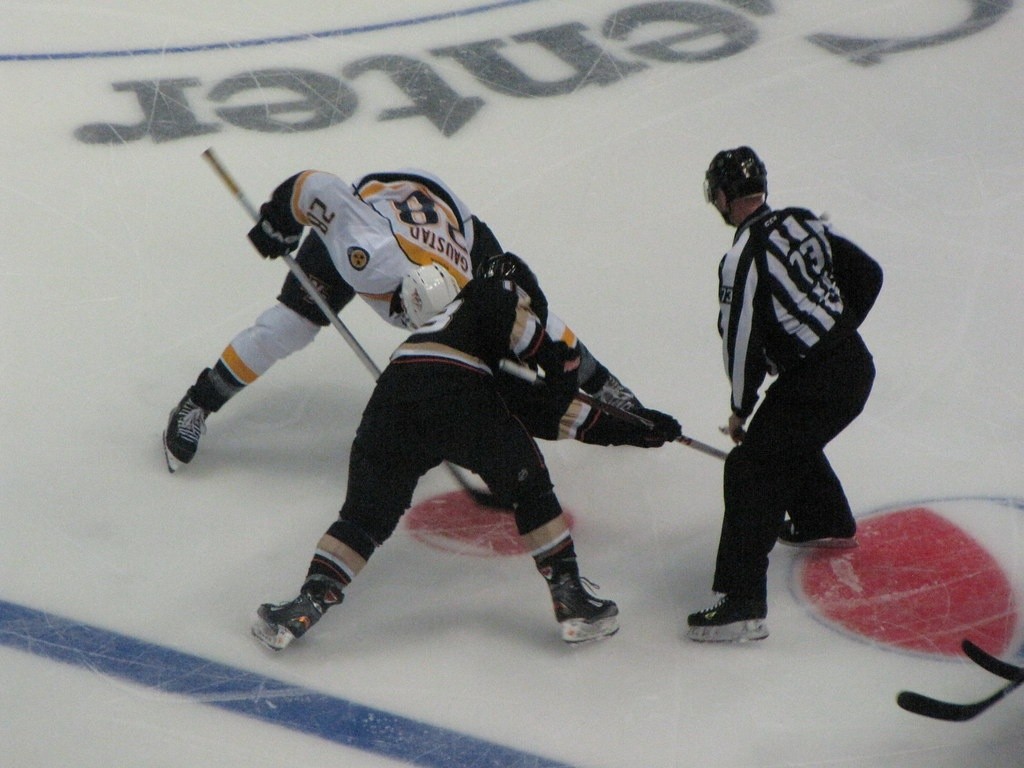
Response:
[475,250,538,291]
[706,146,766,202]
[403,262,461,329]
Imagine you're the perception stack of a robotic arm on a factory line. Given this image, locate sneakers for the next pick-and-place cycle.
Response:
[589,373,644,413]
[537,558,619,642]
[776,519,860,547]
[162,388,211,473]
[687,592,770,641]
[251,576,344,650]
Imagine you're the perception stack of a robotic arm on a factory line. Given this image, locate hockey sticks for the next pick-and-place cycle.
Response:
[201,147,509,511]
[498,358,728,461]
[898,639,1024,722]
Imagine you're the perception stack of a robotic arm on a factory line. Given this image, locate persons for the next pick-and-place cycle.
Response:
[247,254,683,651]
[160,167,507,471]
[687,145,884,642]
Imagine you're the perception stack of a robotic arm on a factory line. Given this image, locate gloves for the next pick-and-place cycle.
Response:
[610,409,681,447]
[248,200,303,260]
[539,341,580,397]
